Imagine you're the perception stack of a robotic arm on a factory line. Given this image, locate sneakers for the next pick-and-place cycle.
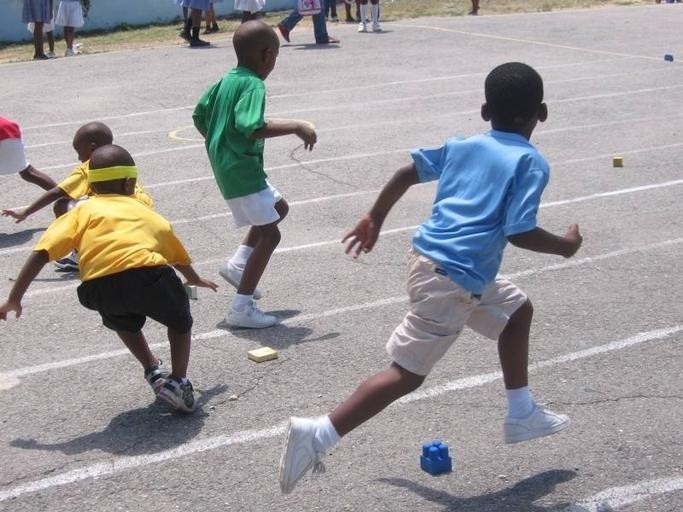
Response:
[218,260,262,299]
[145,359,171,394]
[155,374,196,414]
[65,48,77,55]
[372,23,381,31]
[277,23,290,42]
[190,40,209,46]
[178,29,190,42]
[207,28,219,33]
[358,23,367,32]
[52,248,79,271]
[316,37,339,44]
[505,405,569,444]
[47,51,59,57]
[225,300,276,328]
[278,416,325,494]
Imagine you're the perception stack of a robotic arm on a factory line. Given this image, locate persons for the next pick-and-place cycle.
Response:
[469,0,478,16]
[322,0,338,23]
[175,6,193,31]
[26,10,57,61]
[200,0,220,35]
[192,20,317,330]
[344,0,369,23]
[54,0,90,58]
[233,0,265,25]
[277,62,583,494]
[175,0,211,47]
[358,0,382,33]
[0,114,58,191]
[0,121,155,273]
[0,144,219,415]
[277,0,340,45]
[20,0,53,61]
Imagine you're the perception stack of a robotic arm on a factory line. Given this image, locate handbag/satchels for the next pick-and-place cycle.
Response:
[296,0,321,16]
